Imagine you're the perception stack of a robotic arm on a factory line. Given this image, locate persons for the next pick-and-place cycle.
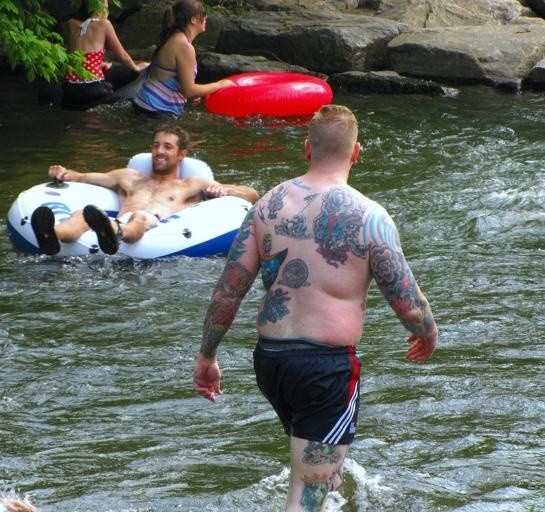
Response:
[193,103,438,511]
[30,124,259,257]
[132,0,233,119]
[60,0,151,111]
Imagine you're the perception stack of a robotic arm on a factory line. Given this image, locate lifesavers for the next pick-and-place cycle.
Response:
[204,72,333,118]
[8,153,254,264]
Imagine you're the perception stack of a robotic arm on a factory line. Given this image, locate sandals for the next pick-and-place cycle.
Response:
[82,203,123,255]
[30,205,62,256]
